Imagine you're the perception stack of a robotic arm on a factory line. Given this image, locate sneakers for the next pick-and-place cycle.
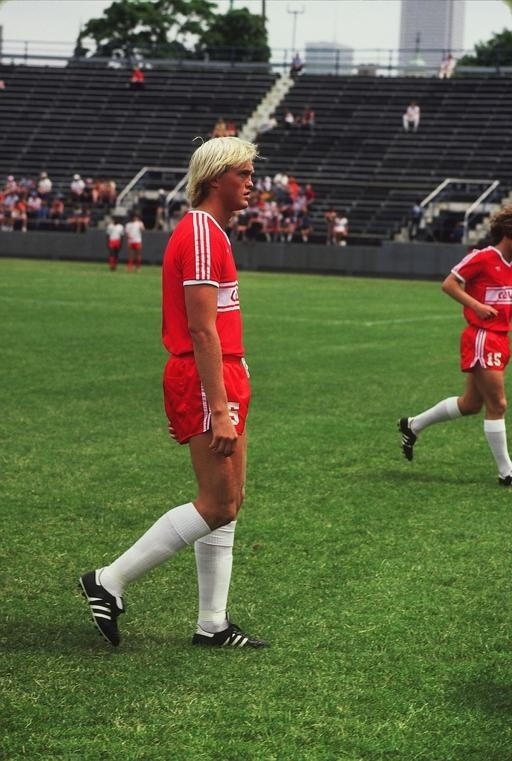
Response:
[77,566,125,649]
[397,415,420,463]
[498,474,512,486]
[190,617,271,649]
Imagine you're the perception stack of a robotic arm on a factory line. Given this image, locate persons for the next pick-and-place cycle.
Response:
[439,54,457,79]
[106,213,145,272]
[258,105,314,135]
[289,52,305,77]
[226,172,316,243]
[0,79,6,91]
[395,202,512,486]
[76,135,277,651]
[325,206,349,246]
[212,118,239,138]
[154,189,190,231]
[0,171,117,232]
[441,57,449,71]
[125,65,145,89]
[410,200,424,240]
[450,221,464,244]
[401,100,420,133]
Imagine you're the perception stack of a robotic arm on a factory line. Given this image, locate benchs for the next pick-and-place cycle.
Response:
[1,62,511,244]
